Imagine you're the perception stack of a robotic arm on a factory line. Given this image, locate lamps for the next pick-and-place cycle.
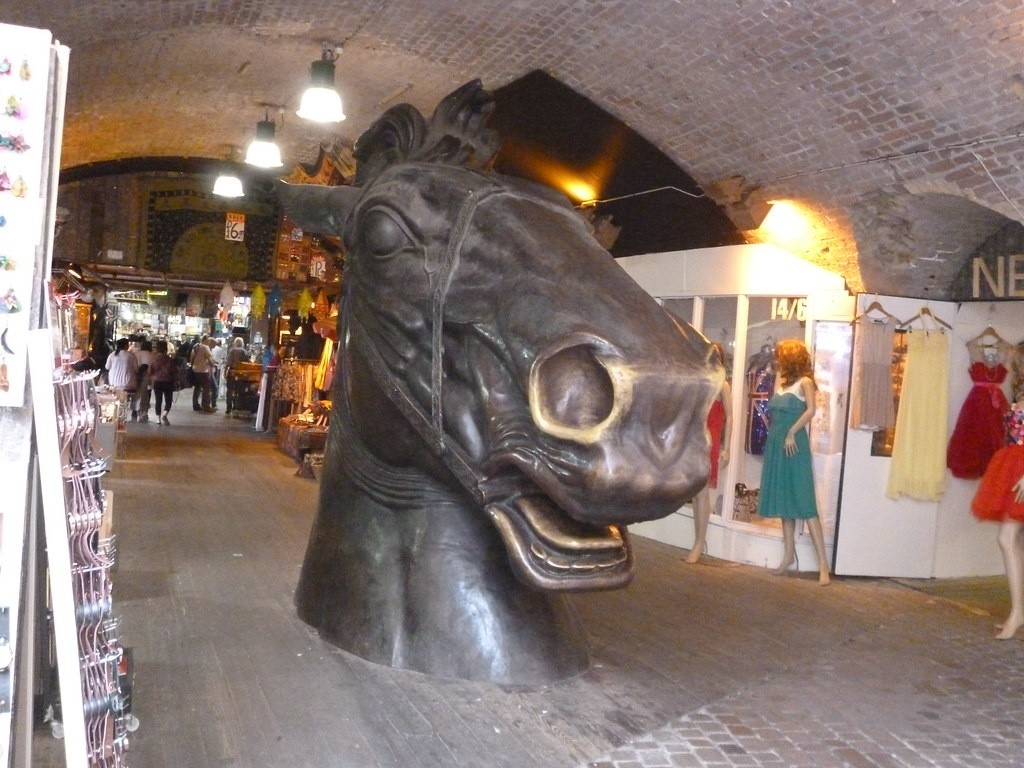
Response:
[212,143,245,198]
[295,40,346,122]
[243,102,286,168]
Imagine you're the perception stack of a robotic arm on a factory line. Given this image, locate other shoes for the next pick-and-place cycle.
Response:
[162,415,169,426]
[203,406,216,413]
[142,415,148,422]
[157,420,161,424]
[194,407,202,411]
[213,406,218,410]
[226,410,231,414]
[128,418,137,423]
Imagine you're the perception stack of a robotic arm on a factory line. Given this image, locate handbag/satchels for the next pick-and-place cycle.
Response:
[187,368,194,383]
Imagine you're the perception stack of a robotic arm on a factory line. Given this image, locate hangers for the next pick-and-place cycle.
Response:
[965,310,1016,351]
[747,344,774,375]
[849,293,902,326]
[900,292,953,331]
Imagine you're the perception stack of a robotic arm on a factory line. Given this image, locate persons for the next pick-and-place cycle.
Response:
[682,342,732,564]
[757,338,832,586]
[105,333,249,426]
[969,341,1024,640]
